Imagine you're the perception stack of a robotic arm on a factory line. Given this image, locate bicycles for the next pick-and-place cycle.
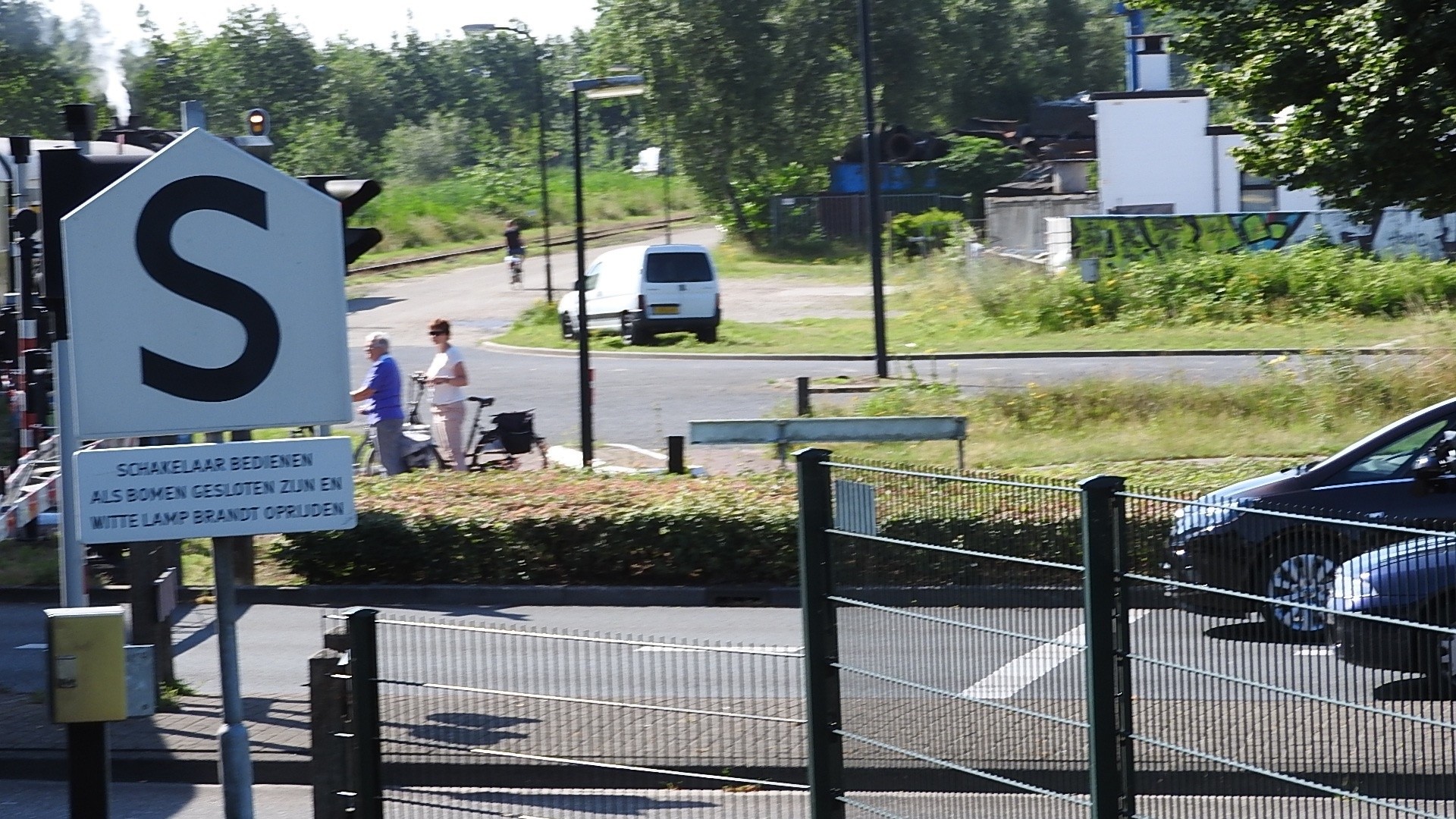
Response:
[348,372,548,472]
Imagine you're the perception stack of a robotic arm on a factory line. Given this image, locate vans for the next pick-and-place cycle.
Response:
[559,244,721,347]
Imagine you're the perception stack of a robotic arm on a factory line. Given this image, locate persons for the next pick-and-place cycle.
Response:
[348,330,412,474]
[503,219,526,269]
[412,317,475,473]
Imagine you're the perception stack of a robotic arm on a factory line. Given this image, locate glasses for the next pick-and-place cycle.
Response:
[429,331,445,336]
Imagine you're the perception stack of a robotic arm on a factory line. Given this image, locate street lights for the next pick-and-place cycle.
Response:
[567,75,651,464]
[462,23,554,306]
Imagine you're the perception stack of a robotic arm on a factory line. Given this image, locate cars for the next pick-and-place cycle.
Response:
[1168,397,1455,683]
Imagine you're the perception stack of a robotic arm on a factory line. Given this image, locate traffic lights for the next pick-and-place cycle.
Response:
[295,168,380,284]
